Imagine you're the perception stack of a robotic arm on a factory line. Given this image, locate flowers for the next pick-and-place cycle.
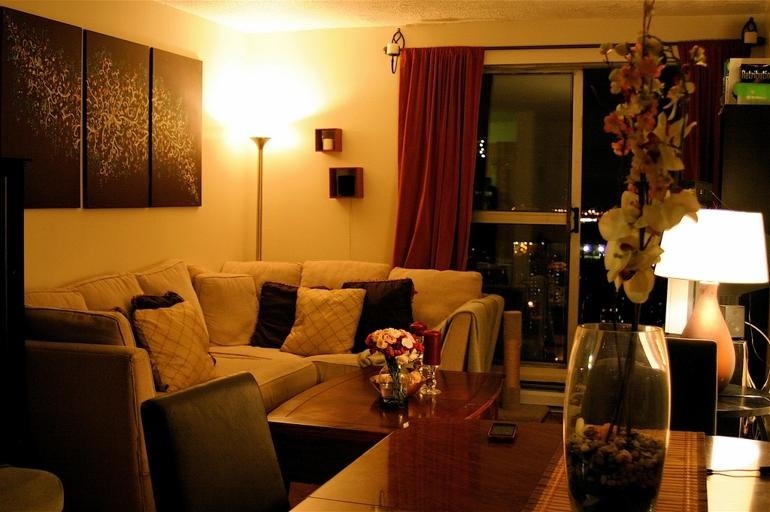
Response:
[598,0,709,443]
[364,327,423,364]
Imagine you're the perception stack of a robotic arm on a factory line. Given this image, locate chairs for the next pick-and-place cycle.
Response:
[141,371,291,512]
[579,332,717,436]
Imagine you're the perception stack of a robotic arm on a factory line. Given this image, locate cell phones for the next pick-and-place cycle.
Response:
[487,422,519,441]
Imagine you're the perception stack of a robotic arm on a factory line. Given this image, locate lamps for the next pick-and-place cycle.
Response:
[240,122,287,262]
[654,209,768,396]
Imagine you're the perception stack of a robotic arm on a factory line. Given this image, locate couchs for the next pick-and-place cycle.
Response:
[23,259,504,512]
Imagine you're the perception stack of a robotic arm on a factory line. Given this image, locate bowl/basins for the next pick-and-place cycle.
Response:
[369,373,426,399]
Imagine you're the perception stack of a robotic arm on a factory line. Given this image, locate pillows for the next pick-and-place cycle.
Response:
[279,286,367,356]
[130,291,215,365]
[341,276,416,355]
[249,281,331,350]
[134,300,223,393]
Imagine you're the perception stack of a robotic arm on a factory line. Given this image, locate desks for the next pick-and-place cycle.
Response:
[287,418,770,512]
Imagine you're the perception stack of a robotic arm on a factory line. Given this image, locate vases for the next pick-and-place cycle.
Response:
[378,368,410,409]
[562,323,672,512]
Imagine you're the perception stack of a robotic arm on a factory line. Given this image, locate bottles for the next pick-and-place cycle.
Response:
[742,16,759,44]
